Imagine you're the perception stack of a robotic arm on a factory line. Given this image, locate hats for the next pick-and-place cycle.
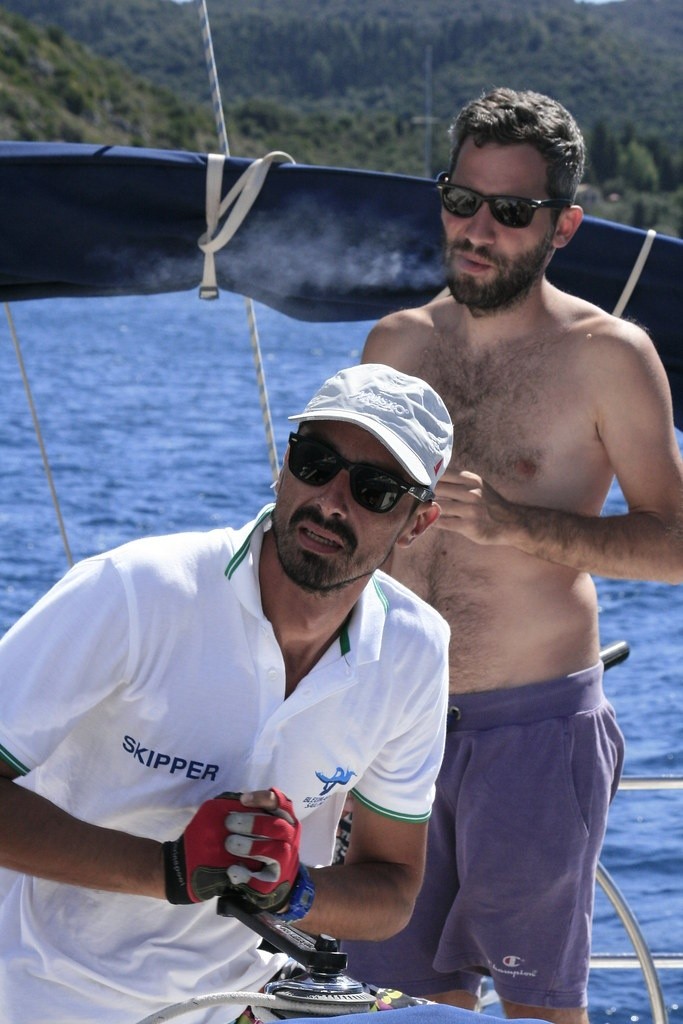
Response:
[288,363,454,493]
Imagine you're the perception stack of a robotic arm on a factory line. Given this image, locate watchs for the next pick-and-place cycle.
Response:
[275,862,316,924]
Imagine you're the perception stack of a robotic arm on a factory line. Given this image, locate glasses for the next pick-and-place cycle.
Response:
[435,171,573,228]
[289,431,434,513]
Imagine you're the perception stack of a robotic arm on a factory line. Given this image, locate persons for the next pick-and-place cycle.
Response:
[333,85,683,1024]
[0,361,453,1024]
